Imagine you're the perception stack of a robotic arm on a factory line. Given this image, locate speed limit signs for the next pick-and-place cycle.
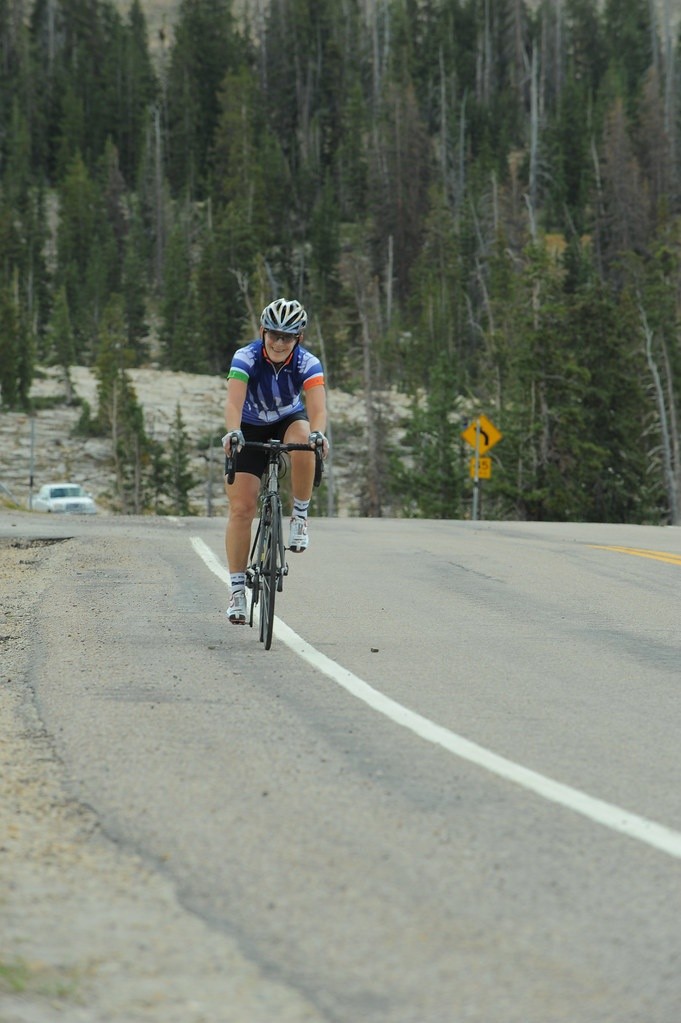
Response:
[472,458,491,480]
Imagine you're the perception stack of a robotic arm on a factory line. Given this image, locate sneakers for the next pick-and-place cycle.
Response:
[226,585,247,623]
[289,516,310,554]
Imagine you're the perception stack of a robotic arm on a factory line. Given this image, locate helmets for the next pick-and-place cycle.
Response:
[260,298,308,334]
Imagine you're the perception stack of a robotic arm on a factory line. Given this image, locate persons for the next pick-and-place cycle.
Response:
[221,298,330,626]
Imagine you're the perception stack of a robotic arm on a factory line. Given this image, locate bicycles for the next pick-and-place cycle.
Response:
[223,430,326,649]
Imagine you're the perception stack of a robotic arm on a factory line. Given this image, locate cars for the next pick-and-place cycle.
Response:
[33,483,99,516]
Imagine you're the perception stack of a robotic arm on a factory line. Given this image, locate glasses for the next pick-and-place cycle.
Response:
[263,328,301,343]
